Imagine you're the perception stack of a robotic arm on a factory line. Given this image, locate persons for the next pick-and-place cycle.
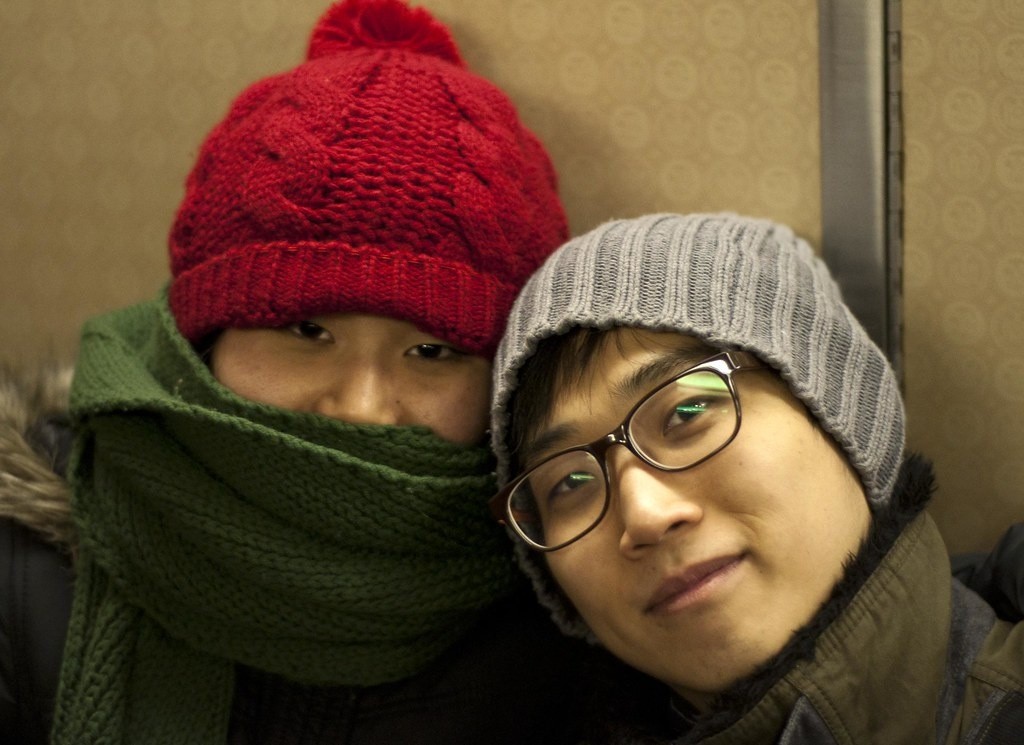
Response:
[493,213,1023,744]
[0,0,1022,745]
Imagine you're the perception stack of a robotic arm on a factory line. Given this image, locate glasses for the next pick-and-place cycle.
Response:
[489,348,773,553]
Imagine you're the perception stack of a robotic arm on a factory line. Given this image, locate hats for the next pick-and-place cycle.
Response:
[490,211,906,642]
[168,0,570,366]
